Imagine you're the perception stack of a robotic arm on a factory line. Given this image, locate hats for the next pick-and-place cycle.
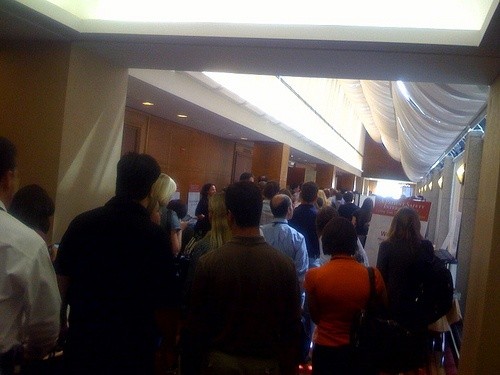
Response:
[166,200,188,219]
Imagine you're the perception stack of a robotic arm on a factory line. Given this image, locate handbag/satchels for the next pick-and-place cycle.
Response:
[181,329,201,375]
[351,268,402,354]
[279,312,312,363]
[415,240,453,323]
[20,351,64,375]
[164,208,190,282]
[354,244,364,263]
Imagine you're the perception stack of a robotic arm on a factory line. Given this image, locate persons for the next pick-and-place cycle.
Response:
[5,184,60,263]
[51,151,178,375]
[0,137,63,375]
[146,173,463,375]
